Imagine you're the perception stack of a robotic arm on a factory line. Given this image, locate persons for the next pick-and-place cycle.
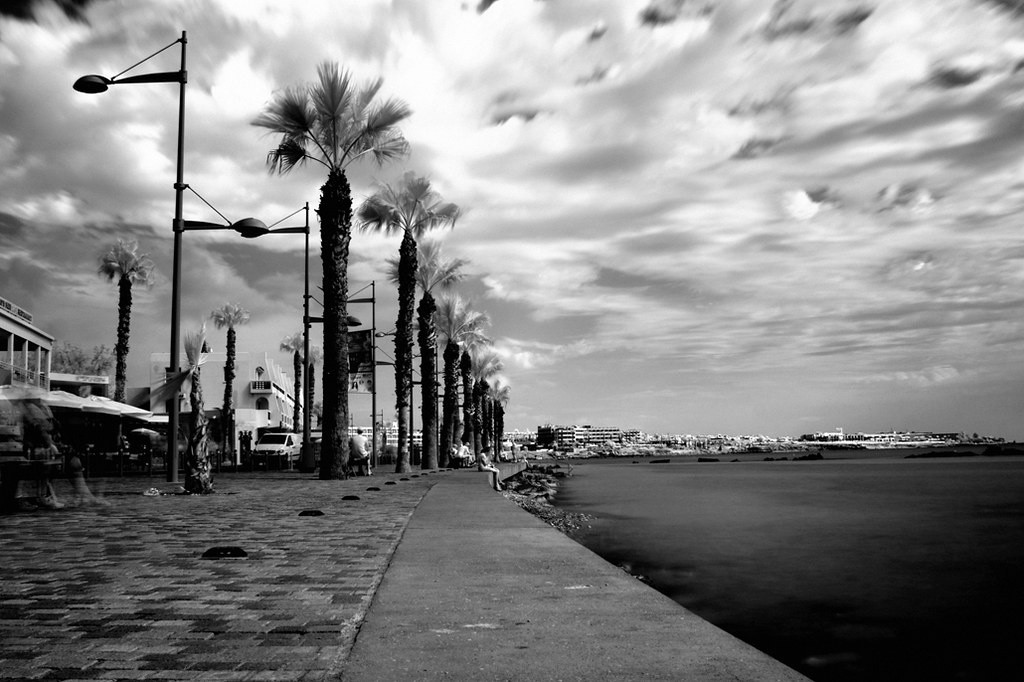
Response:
[510,442,517,462]
[520,446,531,468]
[347,427,374,476]
[477,444,505,492]
[450,438,475,467]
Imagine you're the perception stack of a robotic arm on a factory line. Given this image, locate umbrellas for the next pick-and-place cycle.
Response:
[0,386,153,479]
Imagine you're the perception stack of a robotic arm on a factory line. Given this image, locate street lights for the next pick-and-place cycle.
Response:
[302,281,394,467]
[70,30,270,483]
[173,181,365,474]
[376,324,442,465]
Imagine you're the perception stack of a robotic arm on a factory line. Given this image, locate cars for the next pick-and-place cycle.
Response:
[251,433,304,468]
